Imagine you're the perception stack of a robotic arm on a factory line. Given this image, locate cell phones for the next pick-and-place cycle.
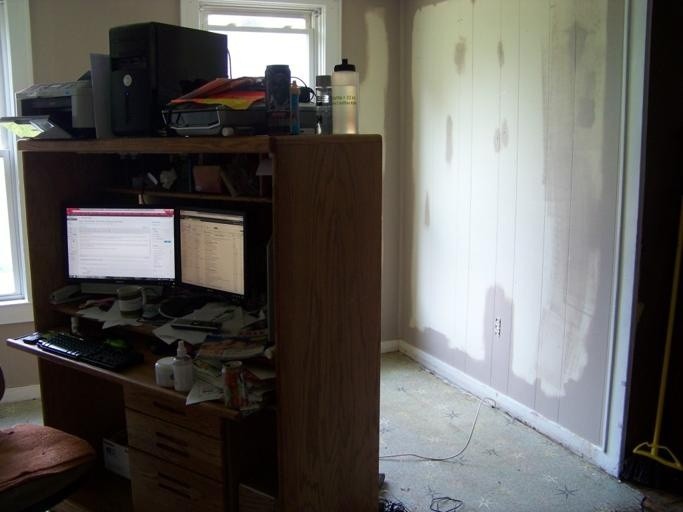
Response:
[23,335,43,344]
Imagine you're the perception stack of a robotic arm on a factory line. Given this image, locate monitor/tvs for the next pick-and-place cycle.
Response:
[178,206,252,308]
[62,203,178,286]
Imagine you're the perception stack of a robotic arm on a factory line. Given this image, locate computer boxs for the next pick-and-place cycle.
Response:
[108,21,228,135]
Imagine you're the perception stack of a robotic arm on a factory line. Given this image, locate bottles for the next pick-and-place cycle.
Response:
[172,340,194,394]
[331,58,360,135]
[264,63,291,135]
[221,360,247,409]
[314,74,332,137]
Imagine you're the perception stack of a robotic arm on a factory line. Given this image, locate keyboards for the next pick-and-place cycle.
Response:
[37,332,145,374]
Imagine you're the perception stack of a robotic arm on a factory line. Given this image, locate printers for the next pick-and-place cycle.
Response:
[0,70,96,140]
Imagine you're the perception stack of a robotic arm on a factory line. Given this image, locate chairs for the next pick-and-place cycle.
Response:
[0,365,103,512]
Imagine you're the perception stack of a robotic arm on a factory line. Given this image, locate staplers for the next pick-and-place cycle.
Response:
[47,284,87,305]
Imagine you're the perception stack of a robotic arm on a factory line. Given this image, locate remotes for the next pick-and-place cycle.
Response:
[170,317,223,332]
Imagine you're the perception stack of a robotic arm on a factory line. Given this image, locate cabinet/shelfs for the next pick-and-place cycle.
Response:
[6,133,384,512]
